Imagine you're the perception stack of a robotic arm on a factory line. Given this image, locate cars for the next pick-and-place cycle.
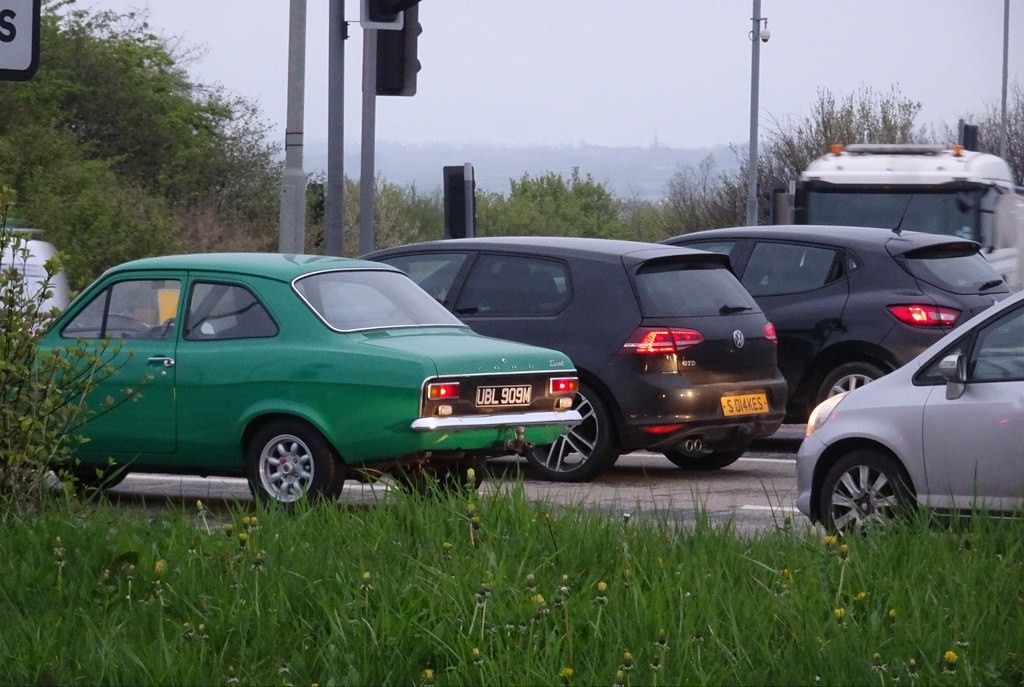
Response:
[653,224,1015,419]
[354,235,791,488]
[26,253,580,520]
[792,285,1023,542]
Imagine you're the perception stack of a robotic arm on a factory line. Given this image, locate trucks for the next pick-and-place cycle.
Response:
[792,143,1016,290]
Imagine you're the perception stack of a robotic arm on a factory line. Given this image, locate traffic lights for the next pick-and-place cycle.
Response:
[763,187,786,225]
